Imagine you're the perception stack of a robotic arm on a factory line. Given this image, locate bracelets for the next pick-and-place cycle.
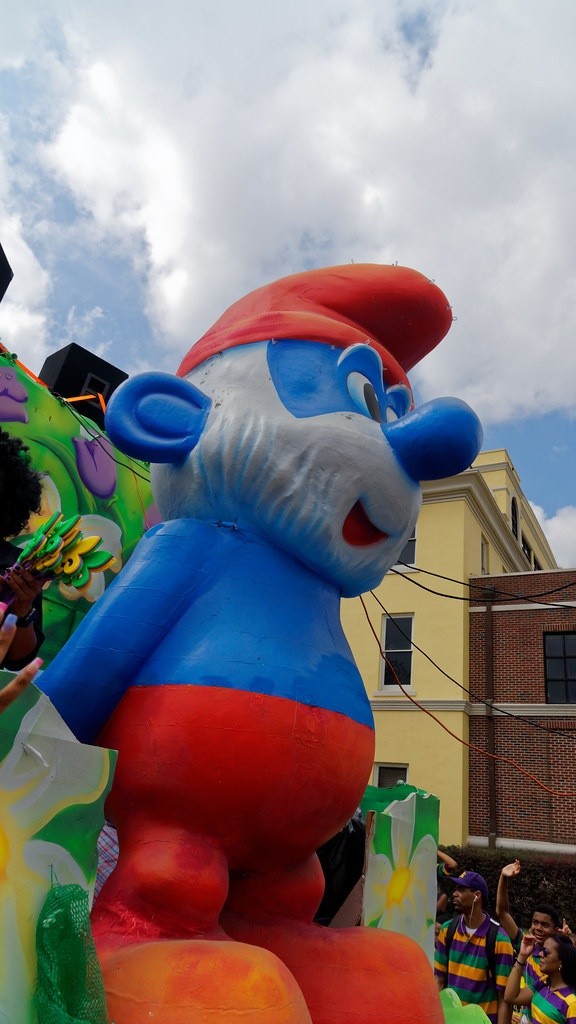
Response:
[9,601,37,628]
[515,958,526,966]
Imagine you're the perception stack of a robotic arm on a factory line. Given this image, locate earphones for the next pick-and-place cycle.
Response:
[473,896,478,902]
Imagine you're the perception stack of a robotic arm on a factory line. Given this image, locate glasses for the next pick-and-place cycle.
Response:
[540,946,551,958]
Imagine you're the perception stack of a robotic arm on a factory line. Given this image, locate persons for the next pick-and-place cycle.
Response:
[0,602,43,714]
[495,860,576,1024]
[437,849,458,883]
[436,893,448,924]
[0,425,47,671]
[433,871,516,1024]
[503,934,576,1024]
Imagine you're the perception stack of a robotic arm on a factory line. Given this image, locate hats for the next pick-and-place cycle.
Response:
[448,870,488,899]
